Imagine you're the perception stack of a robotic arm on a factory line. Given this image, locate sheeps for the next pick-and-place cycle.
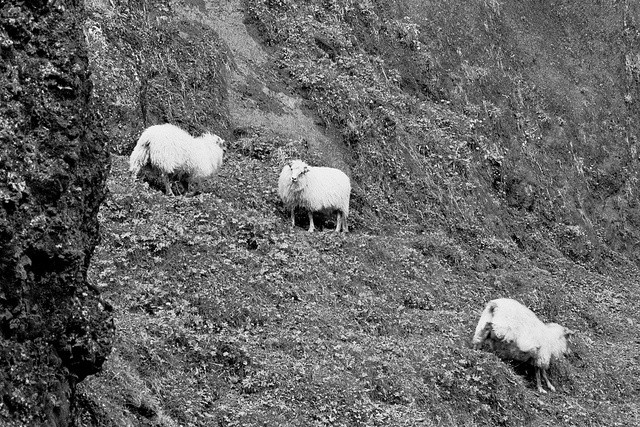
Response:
[471,298,575,394]
[129,123,228,197]
[278,159,351,234]
